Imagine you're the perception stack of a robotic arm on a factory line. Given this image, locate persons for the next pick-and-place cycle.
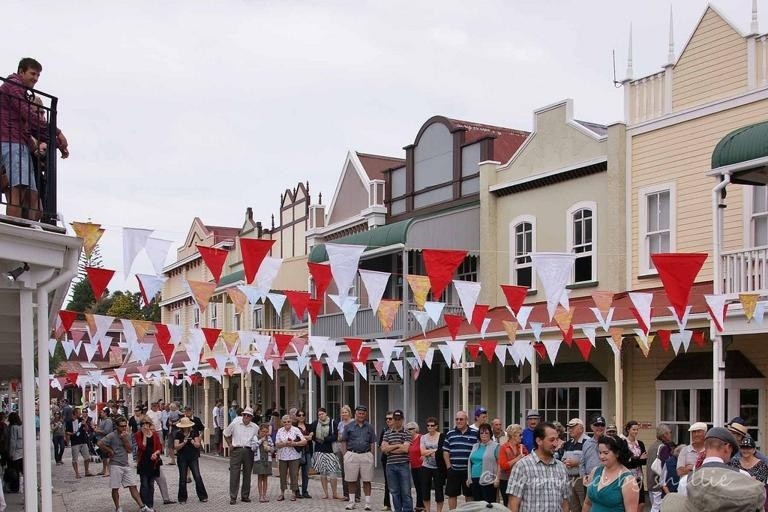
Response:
[0,393,768,512]
[26,94,50,225]
[0,56,69,229]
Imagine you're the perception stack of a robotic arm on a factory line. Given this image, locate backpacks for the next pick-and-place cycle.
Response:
[651,456,663,475]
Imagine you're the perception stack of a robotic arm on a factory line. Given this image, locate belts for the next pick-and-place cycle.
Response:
[347,449,372,454]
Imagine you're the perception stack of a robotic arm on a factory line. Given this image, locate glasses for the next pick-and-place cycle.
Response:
[283,410,466,433]
[34,399,168,441]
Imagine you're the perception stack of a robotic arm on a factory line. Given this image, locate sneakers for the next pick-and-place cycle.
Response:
[140,504,154,512]
[380,505,391,510]
[364,502,372,511]
[229,490,346,505]
[345,500,355,510]
[116,507,122,512]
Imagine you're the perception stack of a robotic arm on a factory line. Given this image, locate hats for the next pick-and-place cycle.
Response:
[704,427,740,451]
[356,404,368,412]
[688,421,708,432]
[728,417,747,437]
[567,416,584,427]
[475,407,488,416]
[590,416,607,425]
[605,424,618,433]
[739,437,756,447]
[654,422,673,436]
[176,417,195,428]
[525,407,542,420]
[242,407,255,417]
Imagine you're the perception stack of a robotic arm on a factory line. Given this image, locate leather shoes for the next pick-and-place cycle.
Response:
[163,500,177,504]
[180,500,186,503]
[201,499,207,502]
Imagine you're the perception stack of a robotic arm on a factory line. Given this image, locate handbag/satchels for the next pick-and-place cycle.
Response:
[331,442,344,453]
